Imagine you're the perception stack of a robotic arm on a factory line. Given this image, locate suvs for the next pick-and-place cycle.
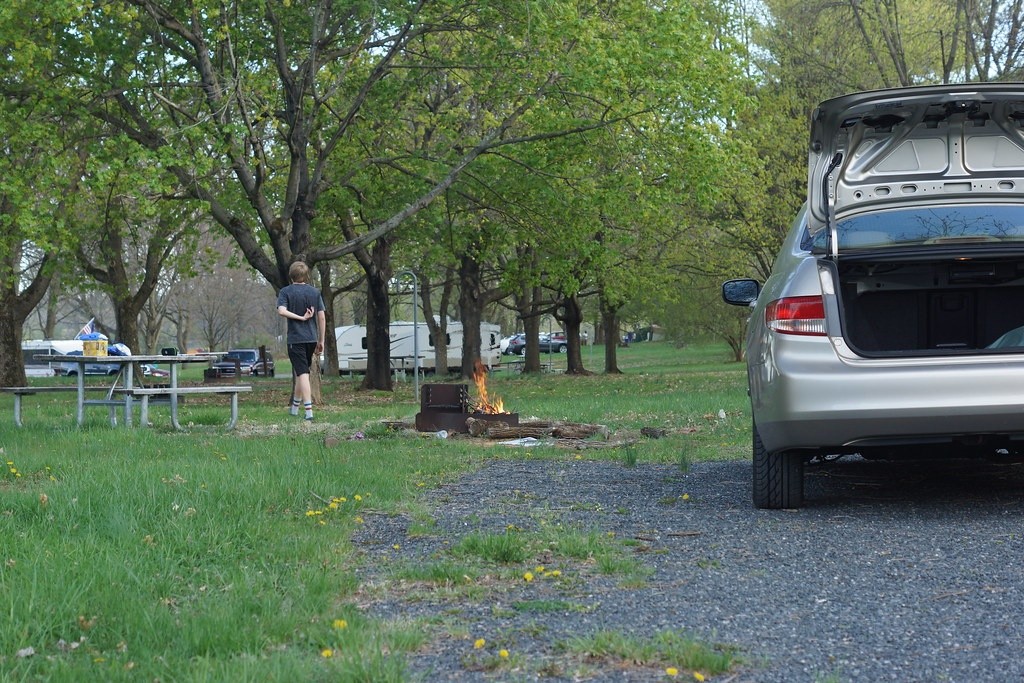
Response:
[500,331,587,356]
[212,348,274,377]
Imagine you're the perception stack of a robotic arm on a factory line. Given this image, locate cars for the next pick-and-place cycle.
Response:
[53,350,170,379]
[720,80,1024,511]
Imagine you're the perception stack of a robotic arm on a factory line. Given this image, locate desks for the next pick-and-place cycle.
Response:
[348,355,428,384]
[30,354,218,432]
[499,361,554,374]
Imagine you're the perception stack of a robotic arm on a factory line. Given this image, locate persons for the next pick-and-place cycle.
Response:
[622,333,626,341]
[583,331,589,345]
[632,332,637,343]
[625,333,628,346]
[629,332,633,342]
[275,262,325,420]
[579,331,582,345]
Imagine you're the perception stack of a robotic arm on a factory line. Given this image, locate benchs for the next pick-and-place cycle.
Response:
[113,386,254,395]
[0,387,125,393]
[391,367,428,369]
[540,364,553,366]
[514,367,546,369]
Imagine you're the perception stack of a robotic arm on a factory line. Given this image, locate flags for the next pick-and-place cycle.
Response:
[83,320,96,333]
